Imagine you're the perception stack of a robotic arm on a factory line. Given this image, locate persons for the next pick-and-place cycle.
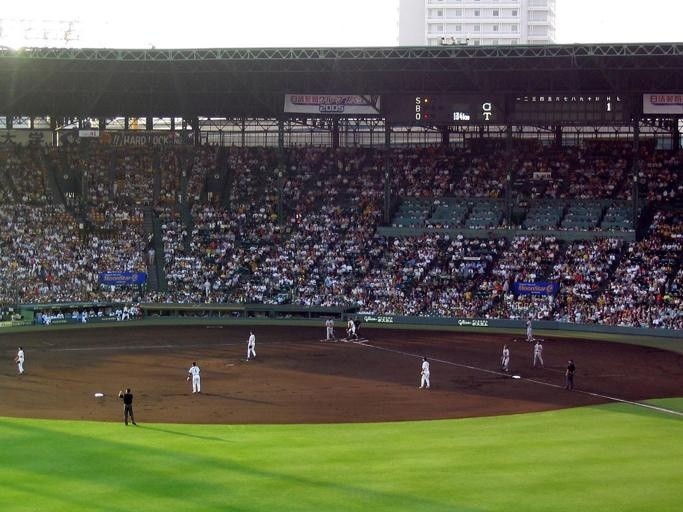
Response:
[565,359,575,389]
[418,356,431,390]
[118,388,137,426]
[532,340,543,367]
[0,125,683,343]
[186,361,201,394]
[499,344,509,373]
[245,330,257,360]
[14,346,25,375]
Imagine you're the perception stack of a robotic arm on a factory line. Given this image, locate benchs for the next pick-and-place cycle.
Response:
[392,194,637,241]
